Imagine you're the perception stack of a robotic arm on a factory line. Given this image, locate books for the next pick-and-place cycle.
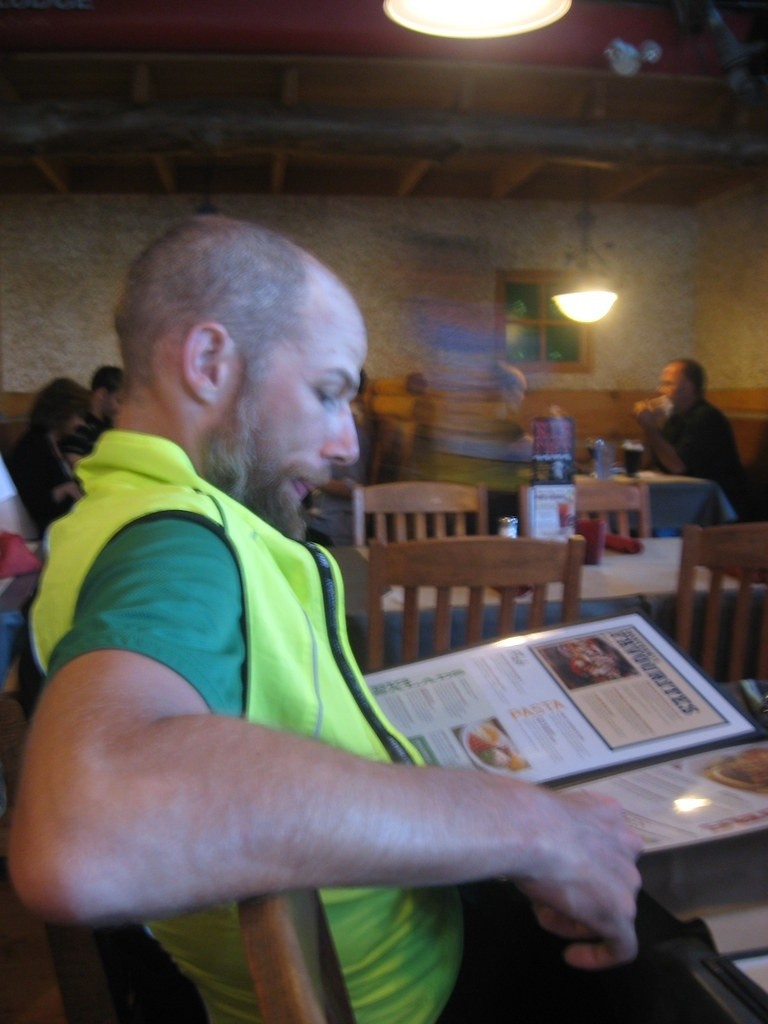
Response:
[362,604,768,881]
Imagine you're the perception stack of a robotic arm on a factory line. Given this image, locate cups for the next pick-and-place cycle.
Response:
[622,444,643,478]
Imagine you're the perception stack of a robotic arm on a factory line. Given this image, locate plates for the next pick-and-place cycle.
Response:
[462,722,520,774]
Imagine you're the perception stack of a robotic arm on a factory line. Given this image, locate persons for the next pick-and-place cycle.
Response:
[631,359,746,539]
[466,361,595,536]
[0,365,128,819]
[300,368,385,547]
[8,215,642,1024]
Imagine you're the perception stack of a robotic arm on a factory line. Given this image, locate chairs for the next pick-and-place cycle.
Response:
[350,481,488,547]
[677,523,768,681]
[366,536,585,672]
[0,697,126,1024]
[238,887,360,1023]
[518,474,650,538]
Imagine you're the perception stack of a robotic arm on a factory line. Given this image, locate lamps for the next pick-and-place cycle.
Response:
[551,173,618,323]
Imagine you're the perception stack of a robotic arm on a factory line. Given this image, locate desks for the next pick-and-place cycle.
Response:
[580,472,736,536]
[323,538,768,672]
[633,681,768,1024]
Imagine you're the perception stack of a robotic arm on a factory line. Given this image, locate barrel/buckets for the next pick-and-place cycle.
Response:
[532,416,576,485]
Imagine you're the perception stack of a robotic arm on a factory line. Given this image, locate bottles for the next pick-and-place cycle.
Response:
[592,436,610,480]
[499,516,519,543]
